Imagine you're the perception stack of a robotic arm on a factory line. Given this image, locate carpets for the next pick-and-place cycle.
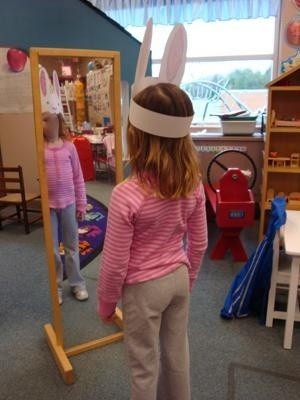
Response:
[57,191,109,281]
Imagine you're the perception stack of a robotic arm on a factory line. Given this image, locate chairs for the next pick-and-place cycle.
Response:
[1,167,41,235]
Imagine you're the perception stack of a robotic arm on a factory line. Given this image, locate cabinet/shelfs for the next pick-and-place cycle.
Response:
[259,60,299,241]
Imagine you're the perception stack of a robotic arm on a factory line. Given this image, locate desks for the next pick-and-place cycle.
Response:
[265,201,299,351]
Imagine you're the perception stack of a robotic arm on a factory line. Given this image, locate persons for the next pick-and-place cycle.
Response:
[42,109,89,306]
[96,82,210,399]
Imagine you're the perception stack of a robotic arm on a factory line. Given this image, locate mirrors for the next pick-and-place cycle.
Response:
[26,45,125,385]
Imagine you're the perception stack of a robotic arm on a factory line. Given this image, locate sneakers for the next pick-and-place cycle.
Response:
[75,289,89,300]
[59,291,63,304]
